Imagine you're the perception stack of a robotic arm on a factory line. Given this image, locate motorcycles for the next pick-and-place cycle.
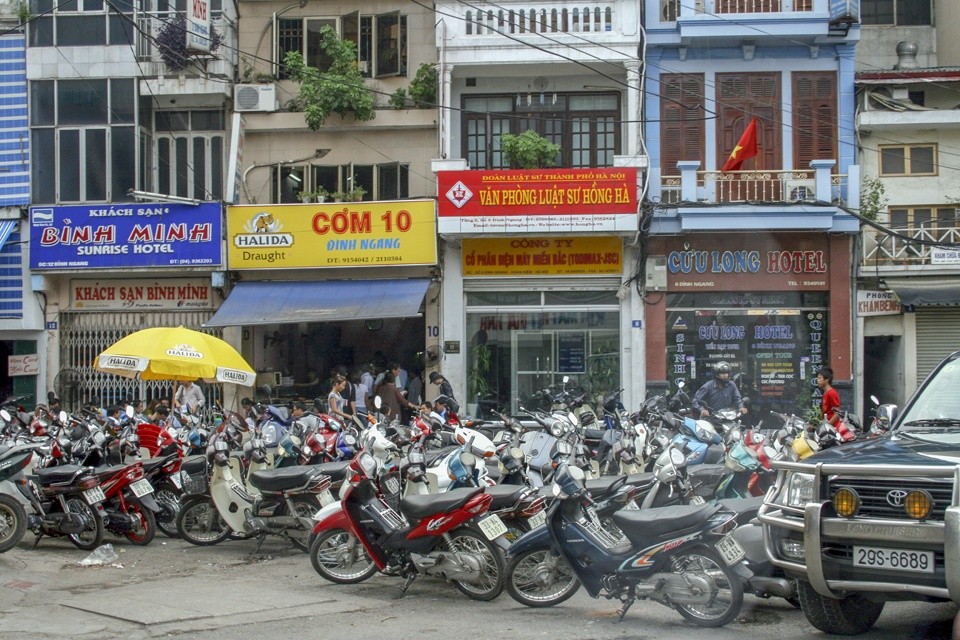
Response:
[308,436,503,602]
[175,426,337,558]
[502,453,756,628]
[0,377,900,609]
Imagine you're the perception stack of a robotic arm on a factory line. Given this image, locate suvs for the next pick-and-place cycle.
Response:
[757,349,960,637]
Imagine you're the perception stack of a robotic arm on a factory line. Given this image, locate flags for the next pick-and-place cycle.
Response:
[721,118,758,176]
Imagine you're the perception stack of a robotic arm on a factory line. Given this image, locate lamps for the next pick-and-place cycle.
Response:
[828,22,852,37]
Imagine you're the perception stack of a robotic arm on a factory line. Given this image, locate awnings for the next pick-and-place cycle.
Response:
[201,279,431,328]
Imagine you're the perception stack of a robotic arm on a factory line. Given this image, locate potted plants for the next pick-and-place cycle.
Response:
[467,344,491,419]
[297,185,367,203]
[409,63,440,109]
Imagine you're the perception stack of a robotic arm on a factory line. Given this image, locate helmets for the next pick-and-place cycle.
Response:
[713,358,733,384]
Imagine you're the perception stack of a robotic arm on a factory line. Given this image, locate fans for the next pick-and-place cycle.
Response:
[419,345,444,367]
[53,369,87,403]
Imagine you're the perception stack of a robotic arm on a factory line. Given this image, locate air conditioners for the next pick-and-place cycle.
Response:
[864,86,908,112]
[785,179,816,201]
[233,83,275,112]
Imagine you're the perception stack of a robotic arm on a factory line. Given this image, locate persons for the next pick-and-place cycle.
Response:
[817,367,840,427]
[692,361,748,416]
[292,362,454,433]
[48,379,262,429]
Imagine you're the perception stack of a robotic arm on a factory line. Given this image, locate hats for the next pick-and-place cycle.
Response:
[337,364,349,377]
[429,370,441,384]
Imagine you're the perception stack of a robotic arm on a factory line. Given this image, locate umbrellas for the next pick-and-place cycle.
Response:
[94,325,257,417]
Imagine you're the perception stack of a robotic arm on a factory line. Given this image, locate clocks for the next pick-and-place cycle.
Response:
[366,319,383,331]
